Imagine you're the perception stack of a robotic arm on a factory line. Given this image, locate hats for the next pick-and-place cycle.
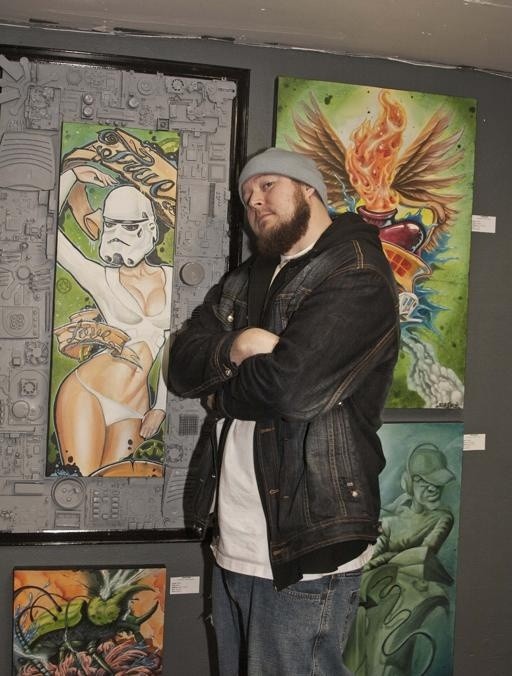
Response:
[238,147,328,209]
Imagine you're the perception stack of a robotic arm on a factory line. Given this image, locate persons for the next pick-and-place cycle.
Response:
[342,438,455,675]
[162,145,401,676]
[54,165,173,476]
